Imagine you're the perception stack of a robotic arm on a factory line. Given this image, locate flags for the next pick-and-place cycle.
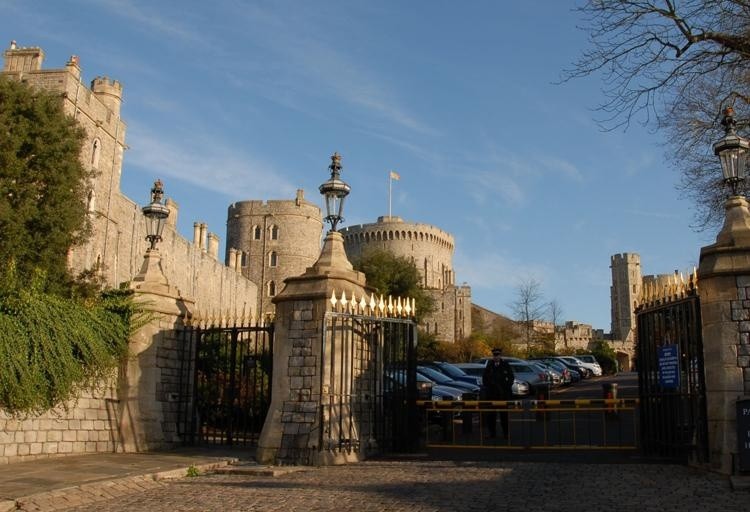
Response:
[391,171,400,180]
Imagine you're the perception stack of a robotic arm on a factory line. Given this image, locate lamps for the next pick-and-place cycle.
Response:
[713,104,750,197]
[140,178,171,250]
[318,151,351,230]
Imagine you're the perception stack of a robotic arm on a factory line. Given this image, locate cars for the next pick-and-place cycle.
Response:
[377,352,602,425]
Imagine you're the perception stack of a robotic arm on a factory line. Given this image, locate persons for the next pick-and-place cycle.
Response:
[482,350,514,444]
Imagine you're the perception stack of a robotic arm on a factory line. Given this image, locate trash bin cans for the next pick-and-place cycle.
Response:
[535,383,550,420]
[737,397,749,474]
[603,383,617,415]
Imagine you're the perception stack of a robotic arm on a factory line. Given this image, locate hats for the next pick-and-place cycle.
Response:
[491,349,502,354]
